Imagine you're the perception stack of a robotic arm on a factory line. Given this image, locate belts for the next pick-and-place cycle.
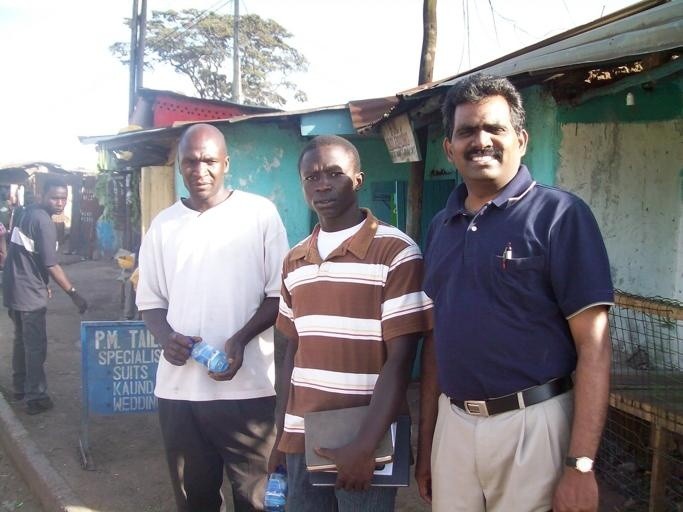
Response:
[438,376,574,415]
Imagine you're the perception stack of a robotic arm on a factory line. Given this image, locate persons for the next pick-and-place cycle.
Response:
[263,135,434,512]
[2,178,88,415]
[413,72,617,512]
[135,123,290,512]
[0,186,13,231]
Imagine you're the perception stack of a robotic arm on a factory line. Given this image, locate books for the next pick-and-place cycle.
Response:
[304,404,410,488]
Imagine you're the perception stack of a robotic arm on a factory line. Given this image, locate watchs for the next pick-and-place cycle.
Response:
[566,454,597,473]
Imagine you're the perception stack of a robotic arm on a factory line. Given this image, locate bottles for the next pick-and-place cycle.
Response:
[188,339,231,373]
[263,464,287,512]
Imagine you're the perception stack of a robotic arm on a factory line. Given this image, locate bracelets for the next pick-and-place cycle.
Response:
[68,288,77,296]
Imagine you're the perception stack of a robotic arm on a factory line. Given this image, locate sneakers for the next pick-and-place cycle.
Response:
[14,392,53,415]
